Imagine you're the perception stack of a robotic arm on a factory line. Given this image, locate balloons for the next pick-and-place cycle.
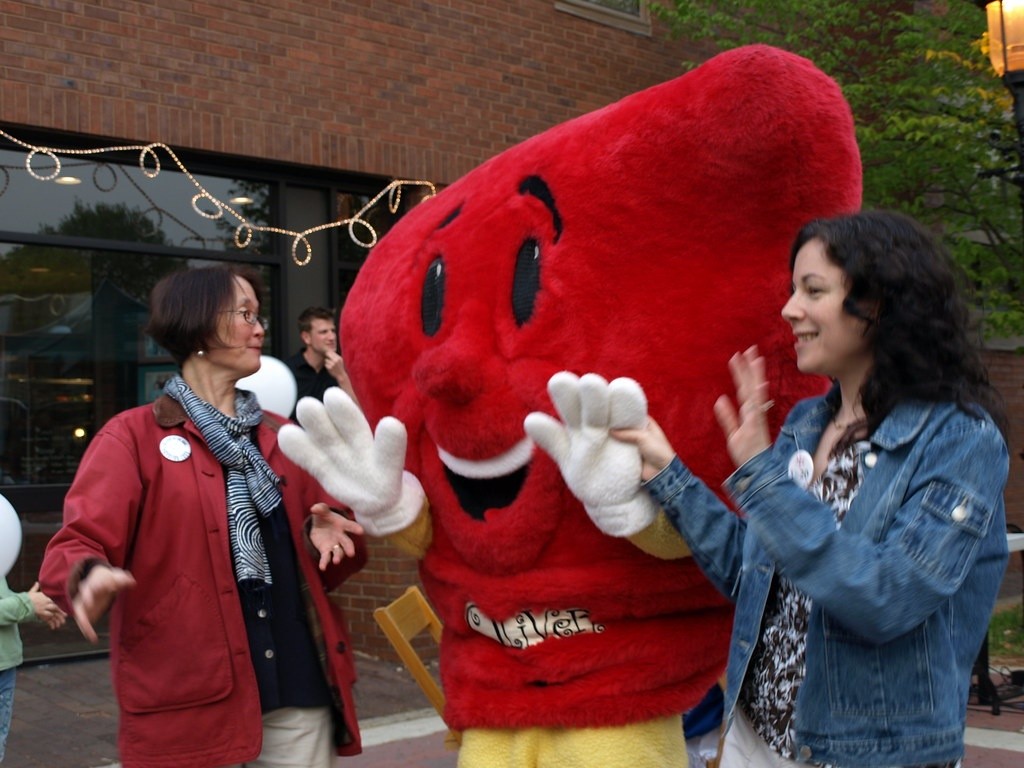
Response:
[234,355,297,419]
[0,495,22,579]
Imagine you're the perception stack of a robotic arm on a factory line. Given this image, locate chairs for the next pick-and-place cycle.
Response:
[375,585,463,750]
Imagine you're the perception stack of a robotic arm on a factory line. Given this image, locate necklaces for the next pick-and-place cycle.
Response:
[829,411,860,431]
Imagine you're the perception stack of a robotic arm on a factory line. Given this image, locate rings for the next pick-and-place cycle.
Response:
[333,544,340,550]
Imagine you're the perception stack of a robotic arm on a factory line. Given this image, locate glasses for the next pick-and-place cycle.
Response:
[218,310,268,330]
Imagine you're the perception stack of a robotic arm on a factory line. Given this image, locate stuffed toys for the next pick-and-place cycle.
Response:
[277,43,862,768]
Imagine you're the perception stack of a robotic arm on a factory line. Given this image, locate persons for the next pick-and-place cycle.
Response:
[609,210,1010,768]
[37,268,365,768]
[288,307,362,428]
[0,575,68,762]
